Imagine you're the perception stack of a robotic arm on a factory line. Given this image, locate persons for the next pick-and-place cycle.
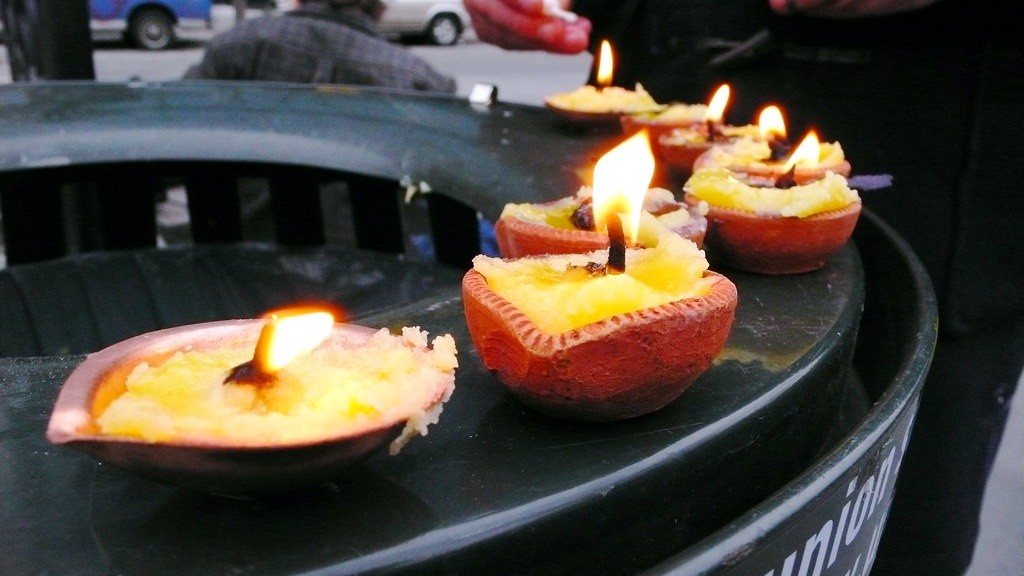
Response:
[571,0,1024,575]
[0,0,96,79]
[182,0,458,252]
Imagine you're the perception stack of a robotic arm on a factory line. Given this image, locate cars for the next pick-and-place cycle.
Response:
[278,0,474,47]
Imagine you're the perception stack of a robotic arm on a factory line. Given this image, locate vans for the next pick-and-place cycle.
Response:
[87,0,211,52]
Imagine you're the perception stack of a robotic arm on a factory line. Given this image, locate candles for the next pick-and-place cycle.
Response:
[659,93,759,201]
[544,39,664,136]
[693,106,853,186]
[622,102,715,162]
[496,164,708,263]
[462,125,738,420]
[47,311,458,485]
[683,129,863,276]
